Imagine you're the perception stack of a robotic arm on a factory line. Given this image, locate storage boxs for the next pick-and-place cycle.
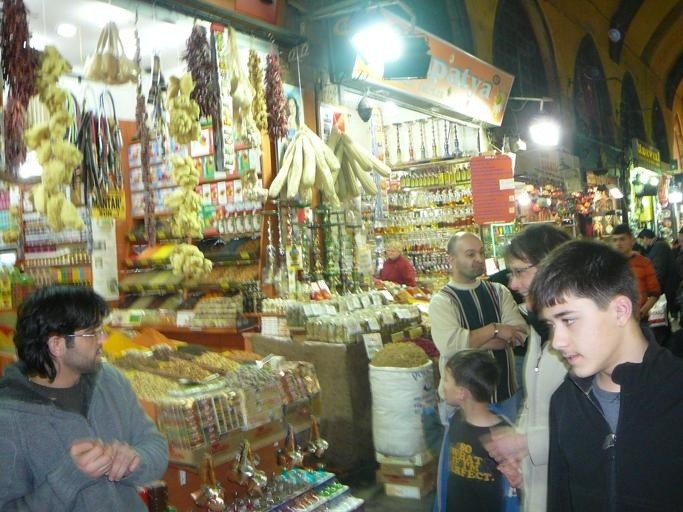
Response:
[373,448,439,502]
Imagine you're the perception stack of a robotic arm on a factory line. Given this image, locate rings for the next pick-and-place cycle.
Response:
[508,340,511,344]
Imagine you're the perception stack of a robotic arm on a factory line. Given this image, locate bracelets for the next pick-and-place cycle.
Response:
[493,322,498,341]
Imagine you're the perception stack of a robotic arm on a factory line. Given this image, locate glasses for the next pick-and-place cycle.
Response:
[68,328,104,338]
[515,264,535,277]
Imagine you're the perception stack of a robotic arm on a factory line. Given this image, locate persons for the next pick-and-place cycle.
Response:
[415,348,522,511]
[378,240,415,285]
[427,232,530,428]
[610,222,682,355]
[0,286,170,512]
[530,240,682,511]
[478,224,573,511]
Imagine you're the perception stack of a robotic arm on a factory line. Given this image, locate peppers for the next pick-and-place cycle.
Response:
[0,1,41,172]
[185,21,221,121]
[264,52,288,138]
